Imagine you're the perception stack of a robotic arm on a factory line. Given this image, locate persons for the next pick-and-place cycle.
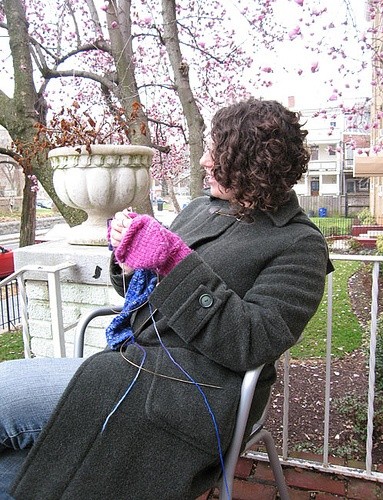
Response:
[0,96,336,499]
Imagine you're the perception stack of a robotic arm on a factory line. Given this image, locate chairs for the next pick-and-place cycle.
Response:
[75,305,291,500]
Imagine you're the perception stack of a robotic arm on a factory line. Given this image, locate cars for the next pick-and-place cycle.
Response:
[0,246,15,280]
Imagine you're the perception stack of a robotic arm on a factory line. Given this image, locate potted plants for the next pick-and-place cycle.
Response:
[45,98,156,247]
